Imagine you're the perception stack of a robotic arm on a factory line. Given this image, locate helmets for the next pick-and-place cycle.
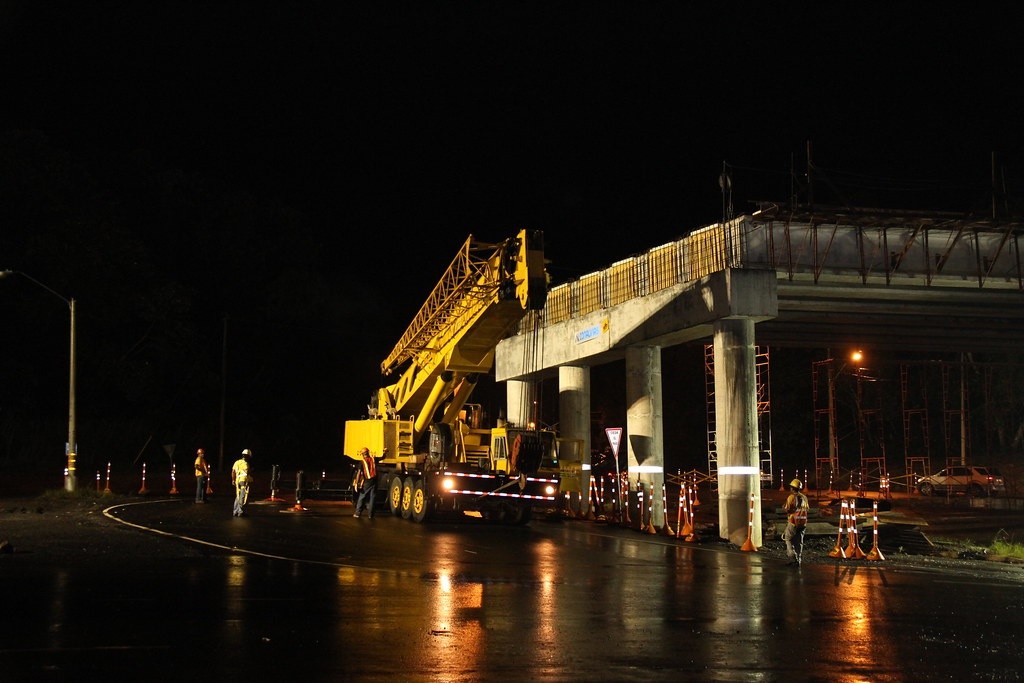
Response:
[789,478,804,491]
[359,448,369,456]
[241,449,252,456]
[196,449,205,454]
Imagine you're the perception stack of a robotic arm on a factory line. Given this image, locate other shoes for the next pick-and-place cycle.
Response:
[195,498,206,504]
[369,515,375,520]
[353,513,360,518]
[238,513,249,519]
[233,511,239,517]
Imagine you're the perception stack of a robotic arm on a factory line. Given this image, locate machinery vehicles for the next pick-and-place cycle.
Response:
[344,227,563,527]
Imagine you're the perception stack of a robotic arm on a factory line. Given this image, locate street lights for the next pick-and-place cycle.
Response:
[1,269,77,492]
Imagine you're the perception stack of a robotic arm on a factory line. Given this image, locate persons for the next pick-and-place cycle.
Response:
[351,447,389,520]
[231,448,253,516]
[779,478,811,567]
[194,448,208,503]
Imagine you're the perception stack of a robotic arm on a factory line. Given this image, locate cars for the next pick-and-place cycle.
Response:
[917,465,1007,498]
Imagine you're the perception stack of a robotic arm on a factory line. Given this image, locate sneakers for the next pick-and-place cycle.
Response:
[794,558,803,567]
[785,555,797,566]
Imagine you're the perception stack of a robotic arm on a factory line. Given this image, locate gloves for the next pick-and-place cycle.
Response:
[383,448,390,455]
[354,483,359,492]
